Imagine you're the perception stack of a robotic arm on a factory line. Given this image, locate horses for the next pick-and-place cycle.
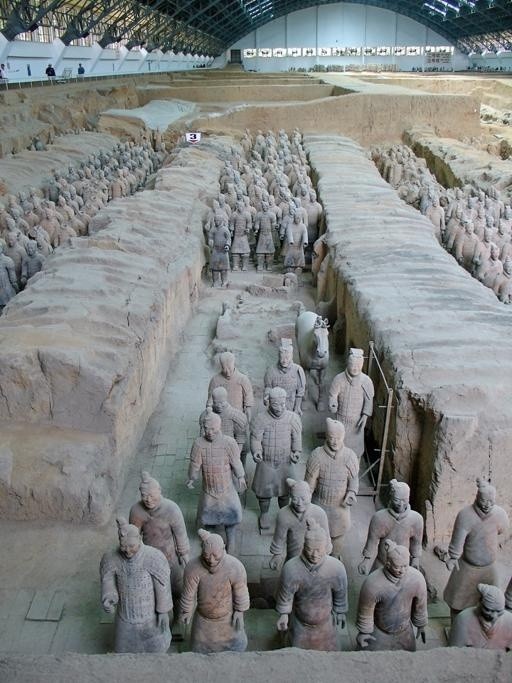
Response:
[294,301,331,413]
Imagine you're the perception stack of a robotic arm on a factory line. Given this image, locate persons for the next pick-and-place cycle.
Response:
[250,388,303,529]
[202,127,324,287]
[276,518,349,650]
[358,479,424,576]
[269,478,332,571]
[97,516,174,650]
[304,418,360,559]
[451,583,512,650]
[355,539,428,650]
[177,528,250,650]
[443,477,511,619]
[263,338,306,417]
[45,63,56,77]
[208,352,253,423]
[327,348,375,460]
[1,120,170,319]
[128,470,190,629]
[0,63,9,78]
[370,145,511,308]
[78,63,84,74]
[199,387,250,495]
[184,408,247,554]
